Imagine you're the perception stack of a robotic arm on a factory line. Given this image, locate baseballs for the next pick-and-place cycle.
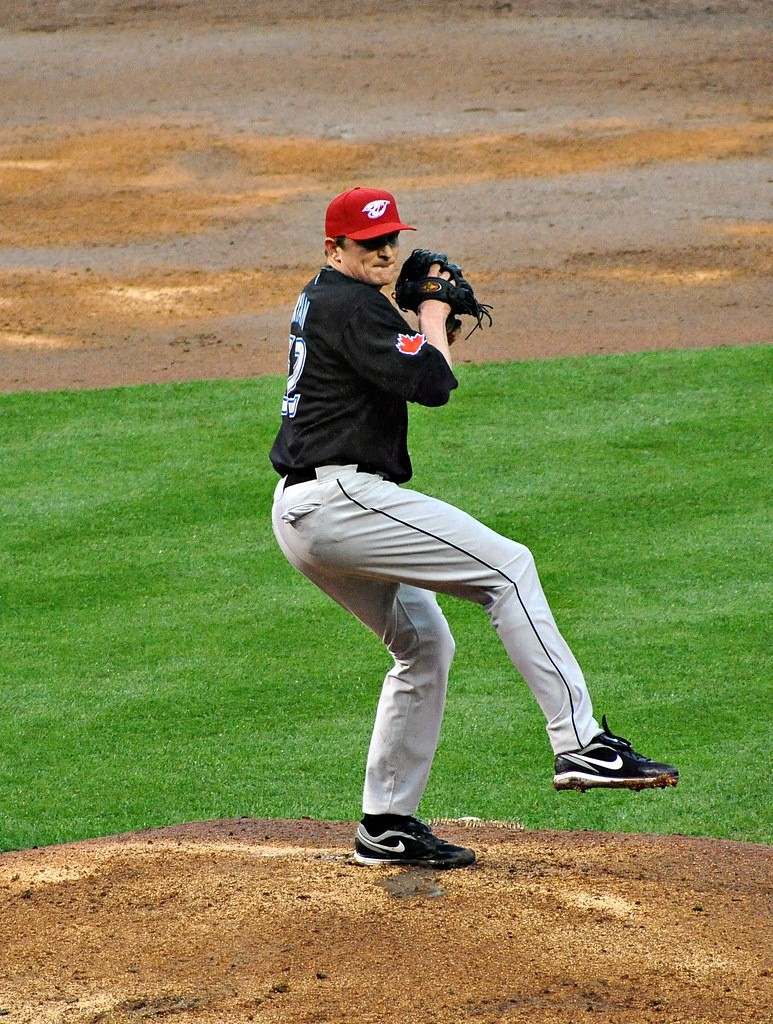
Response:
[445,315,456,333]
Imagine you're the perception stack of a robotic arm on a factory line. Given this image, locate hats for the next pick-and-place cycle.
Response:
[325,186,417,241]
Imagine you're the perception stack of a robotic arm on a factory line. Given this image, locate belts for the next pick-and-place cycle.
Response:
[283,463,377,489]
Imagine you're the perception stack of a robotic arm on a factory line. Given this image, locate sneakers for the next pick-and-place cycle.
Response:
[353,812,475,870]
[553,712,682,792]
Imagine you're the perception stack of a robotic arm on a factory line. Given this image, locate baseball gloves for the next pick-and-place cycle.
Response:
[391,248,494,341]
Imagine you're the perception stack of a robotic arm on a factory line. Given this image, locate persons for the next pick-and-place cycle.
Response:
[270,186,679,870]
